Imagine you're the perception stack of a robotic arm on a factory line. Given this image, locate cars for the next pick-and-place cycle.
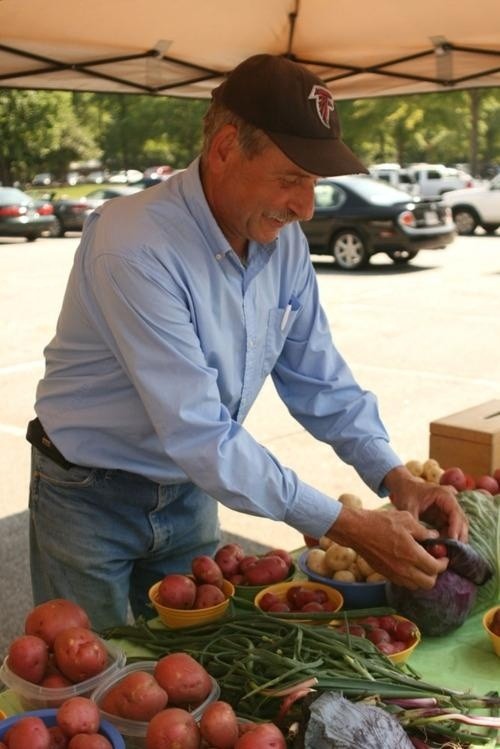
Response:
[293,157,500,268]
[29,156,185,235]
[0,185,55,241]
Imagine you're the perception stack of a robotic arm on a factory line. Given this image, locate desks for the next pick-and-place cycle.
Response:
[0,480,500,748]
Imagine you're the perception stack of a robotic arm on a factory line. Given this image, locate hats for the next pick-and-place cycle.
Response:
[210,52,373,177]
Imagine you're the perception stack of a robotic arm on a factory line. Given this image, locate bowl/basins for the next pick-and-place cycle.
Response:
[4,707,127,748]
[482,606,500,656]
[327,611,421,664]
[233,563,297,590]
[255,581,344,624]
[150,574,235,628]
[297,544,386,606]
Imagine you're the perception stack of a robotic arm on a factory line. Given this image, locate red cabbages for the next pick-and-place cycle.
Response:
[398,538,494,633]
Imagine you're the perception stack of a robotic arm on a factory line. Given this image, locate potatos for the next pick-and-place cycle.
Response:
[217,543,293,585]
[157,555,229,610]
[260,583,335,618]
[144,701,284,749]
[304,535,385,583]
[98,649,208,719]
[405,454,500,496]
[341,614,418,657]
[426,544,447,559]
[7,599,110,689]
[0,697,116,748]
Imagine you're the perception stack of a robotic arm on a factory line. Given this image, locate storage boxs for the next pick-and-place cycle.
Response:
[429,398,500,479]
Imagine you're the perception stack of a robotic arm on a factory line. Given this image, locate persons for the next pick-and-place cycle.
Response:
[27,54,469,641]
